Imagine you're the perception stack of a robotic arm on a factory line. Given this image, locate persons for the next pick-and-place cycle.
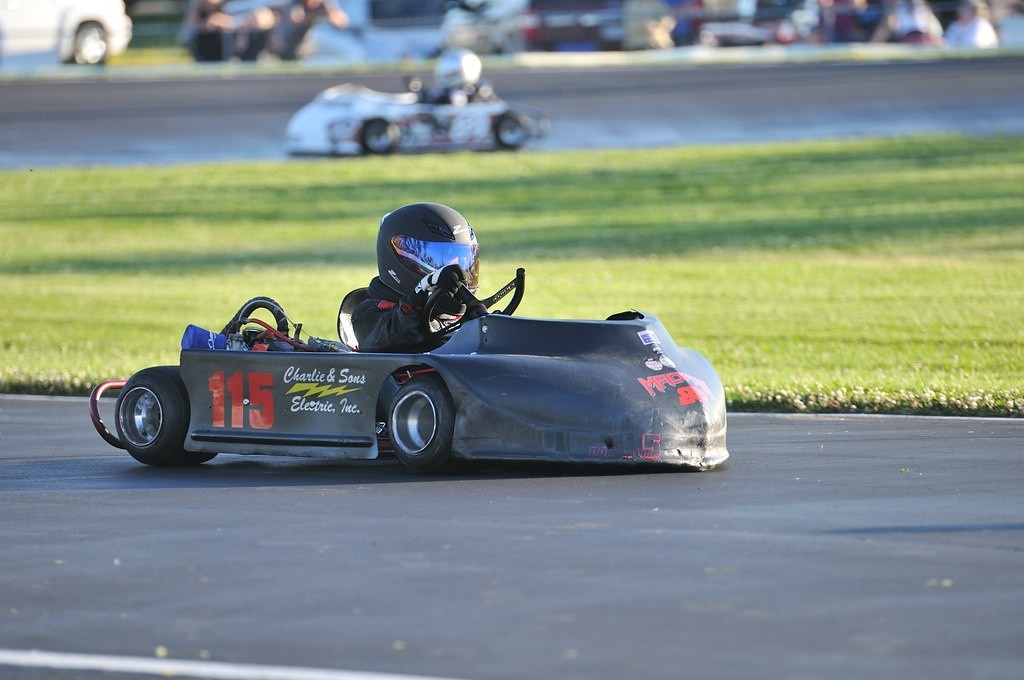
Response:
[176,0,1023,104]
[340,203,482,353]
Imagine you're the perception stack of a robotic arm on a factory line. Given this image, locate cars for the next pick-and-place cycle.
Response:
[416,0,898,61]
[0,0,134,65]
[180,1,370,60]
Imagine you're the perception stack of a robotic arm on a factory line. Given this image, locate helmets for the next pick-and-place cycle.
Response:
[376,201,479,298]
[431,46,481,91]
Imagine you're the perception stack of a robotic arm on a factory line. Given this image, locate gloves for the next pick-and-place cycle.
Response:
[408,264,468,308]
